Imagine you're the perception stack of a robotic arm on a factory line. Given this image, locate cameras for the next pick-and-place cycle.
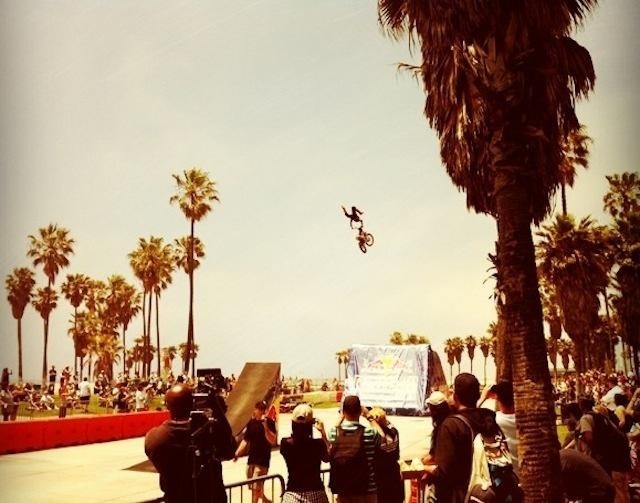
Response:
[312,418,316,424]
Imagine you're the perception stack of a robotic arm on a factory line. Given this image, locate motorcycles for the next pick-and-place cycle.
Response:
[352,226,374,253]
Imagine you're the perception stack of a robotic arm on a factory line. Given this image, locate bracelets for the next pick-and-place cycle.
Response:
[367,415,374,421]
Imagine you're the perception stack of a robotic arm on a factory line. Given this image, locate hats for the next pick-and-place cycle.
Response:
[291,404,312,422]
[426,391,447,405]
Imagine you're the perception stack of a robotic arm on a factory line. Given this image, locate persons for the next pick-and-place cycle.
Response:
[233,399,277,503]
[280,403,333,503]
[342,205,364,231]
[0,364,236,421]
[421,365,639,502]
[369,406,404,502]
[281,374,344,403]
[146,381,240,503]
[327,396,386,503]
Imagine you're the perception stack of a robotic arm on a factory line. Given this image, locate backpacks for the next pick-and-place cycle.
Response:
[598,414,630,472]
[463,432,519,503]
[329,429,368,495]
